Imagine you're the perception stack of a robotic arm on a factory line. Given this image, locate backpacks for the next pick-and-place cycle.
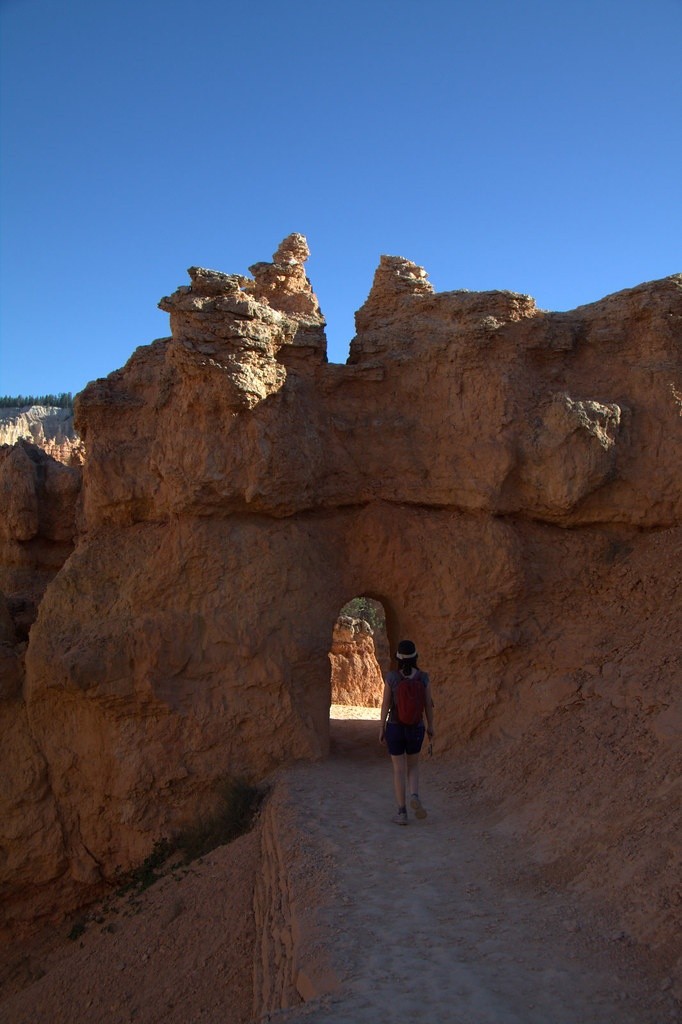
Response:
[393,668,425,728]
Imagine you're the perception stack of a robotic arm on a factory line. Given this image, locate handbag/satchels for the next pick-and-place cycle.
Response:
[385,721,401,741]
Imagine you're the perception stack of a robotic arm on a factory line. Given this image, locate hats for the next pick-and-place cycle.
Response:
[394,640,419,662]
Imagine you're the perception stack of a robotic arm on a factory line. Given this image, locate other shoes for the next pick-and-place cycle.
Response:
[394,813,410,826]
[409,795,428,820]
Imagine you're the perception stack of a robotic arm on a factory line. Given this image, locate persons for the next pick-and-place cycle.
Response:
[379,640,434,824]
